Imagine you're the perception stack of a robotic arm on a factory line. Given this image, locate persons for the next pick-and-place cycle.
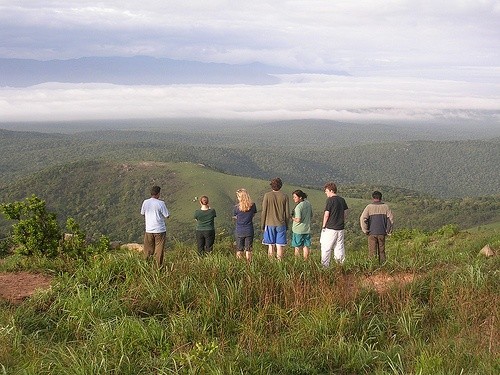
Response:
[321,183,349,266]
[194,195,217,256]
[262,178,290,262]
[141,187,169,266]
[292,190,313,261]
[232,188,257,260]
[360,191,393,263]
